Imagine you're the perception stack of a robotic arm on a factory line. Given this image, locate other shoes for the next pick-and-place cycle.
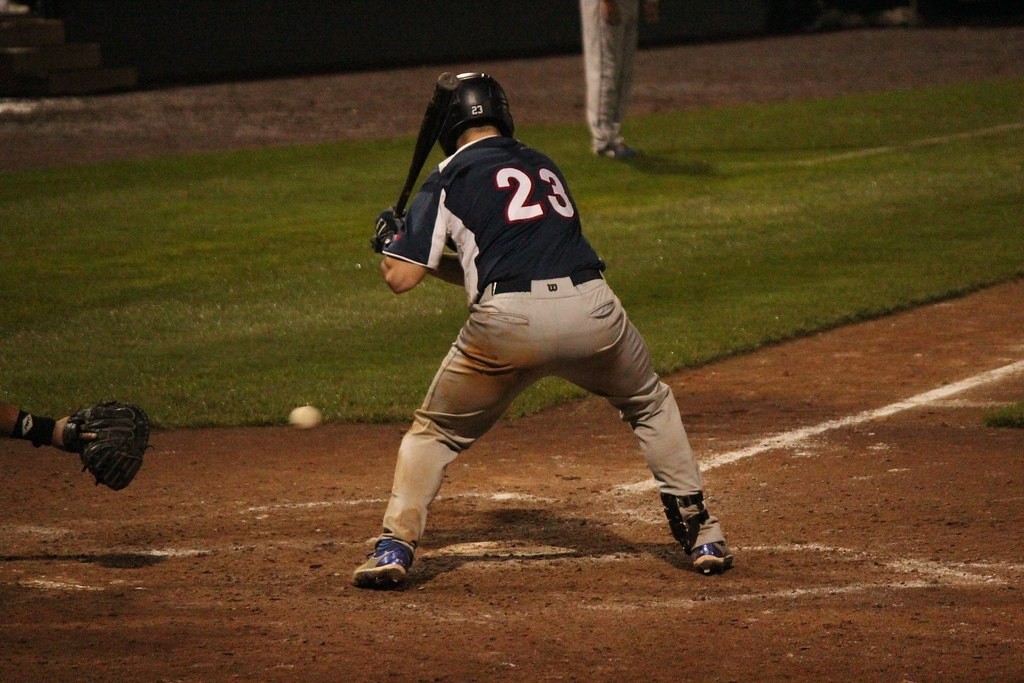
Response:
[593,143,636,158]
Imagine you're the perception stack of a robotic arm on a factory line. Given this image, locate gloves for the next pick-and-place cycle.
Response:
[374,206,408,247]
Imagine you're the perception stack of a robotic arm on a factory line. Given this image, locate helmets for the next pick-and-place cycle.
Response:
[438,72,514,156]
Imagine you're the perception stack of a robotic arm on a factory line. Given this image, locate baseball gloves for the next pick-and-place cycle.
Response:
[85,401,151,491]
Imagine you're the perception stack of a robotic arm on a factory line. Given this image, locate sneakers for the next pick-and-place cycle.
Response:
[352,541,412,587]
[693,541,733,572]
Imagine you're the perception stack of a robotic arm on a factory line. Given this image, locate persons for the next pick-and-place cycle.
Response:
[580,1,637,160]
[355,73,732,585]
[1,400,98,454]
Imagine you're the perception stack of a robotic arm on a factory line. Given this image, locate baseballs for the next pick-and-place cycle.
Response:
[290,405,320,430]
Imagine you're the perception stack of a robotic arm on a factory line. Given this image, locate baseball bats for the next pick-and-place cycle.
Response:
[394,73,460,217]
